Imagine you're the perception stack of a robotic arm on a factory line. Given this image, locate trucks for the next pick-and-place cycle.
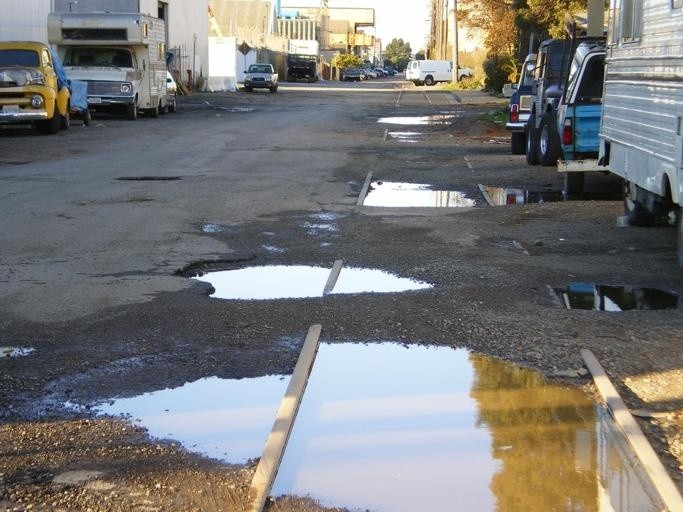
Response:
[46,12,166,119]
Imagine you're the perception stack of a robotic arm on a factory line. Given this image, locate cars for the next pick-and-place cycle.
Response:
[166,69,177,112]
[505,35,606,193]
[243,64,279,92]
[0,40,70,135]
[343,66,398,81]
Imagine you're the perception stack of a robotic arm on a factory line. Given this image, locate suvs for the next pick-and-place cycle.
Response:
[286,53,318,82]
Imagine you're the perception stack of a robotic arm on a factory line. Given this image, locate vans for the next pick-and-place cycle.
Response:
[405,59,475,87]
